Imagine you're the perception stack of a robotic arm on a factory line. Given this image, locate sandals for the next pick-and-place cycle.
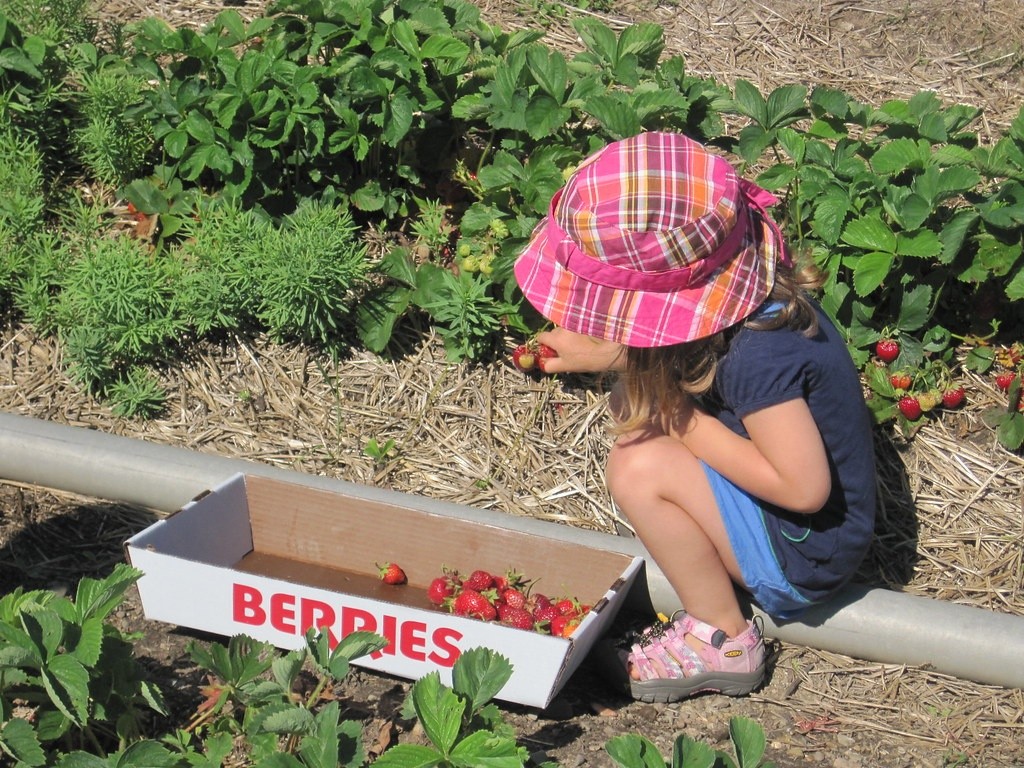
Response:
[612,608,768,703]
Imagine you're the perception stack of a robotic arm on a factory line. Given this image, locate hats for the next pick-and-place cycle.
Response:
[514,131,793,347]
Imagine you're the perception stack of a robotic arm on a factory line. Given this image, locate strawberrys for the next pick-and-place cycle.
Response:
[376,562,595,640]
[877,338,1024,421]
[514,343,559,373]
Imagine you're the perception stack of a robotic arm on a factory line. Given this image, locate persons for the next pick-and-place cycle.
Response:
[513,131,876,704]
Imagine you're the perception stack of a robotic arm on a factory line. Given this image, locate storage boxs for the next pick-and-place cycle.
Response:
[122,475,642,711]
